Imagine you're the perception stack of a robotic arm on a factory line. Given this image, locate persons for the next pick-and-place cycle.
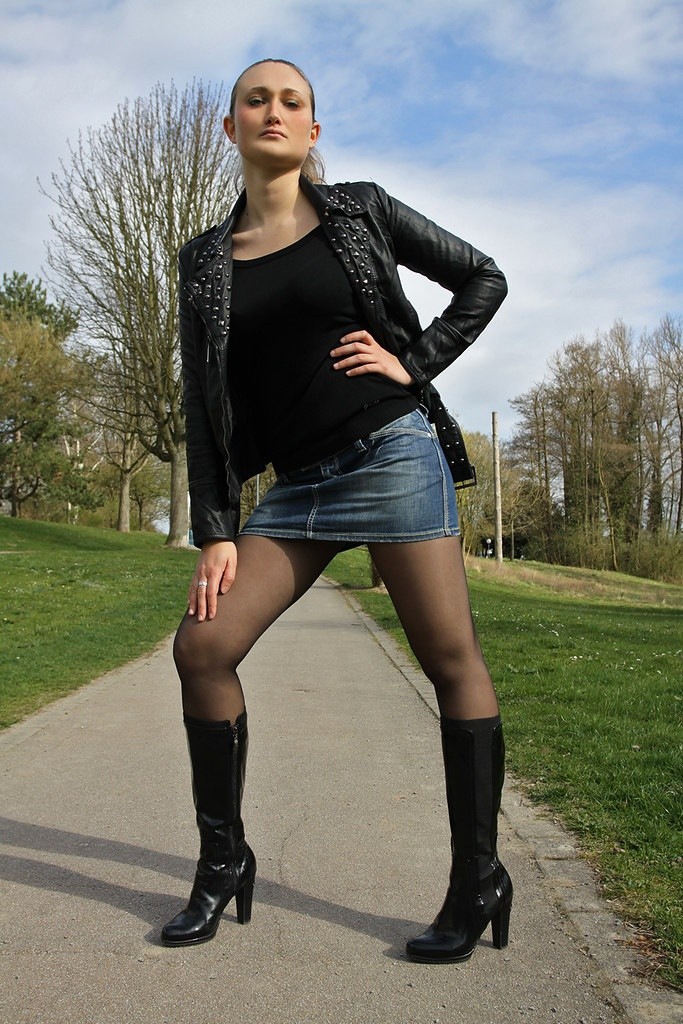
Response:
[160,57,514,965]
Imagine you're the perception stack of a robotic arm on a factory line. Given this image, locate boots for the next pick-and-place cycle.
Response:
[407,713,513,964]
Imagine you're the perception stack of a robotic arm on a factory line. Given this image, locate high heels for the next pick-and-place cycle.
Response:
[161,705,258,948]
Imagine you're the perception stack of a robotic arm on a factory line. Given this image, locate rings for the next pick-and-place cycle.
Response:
[198,582,208,586]
